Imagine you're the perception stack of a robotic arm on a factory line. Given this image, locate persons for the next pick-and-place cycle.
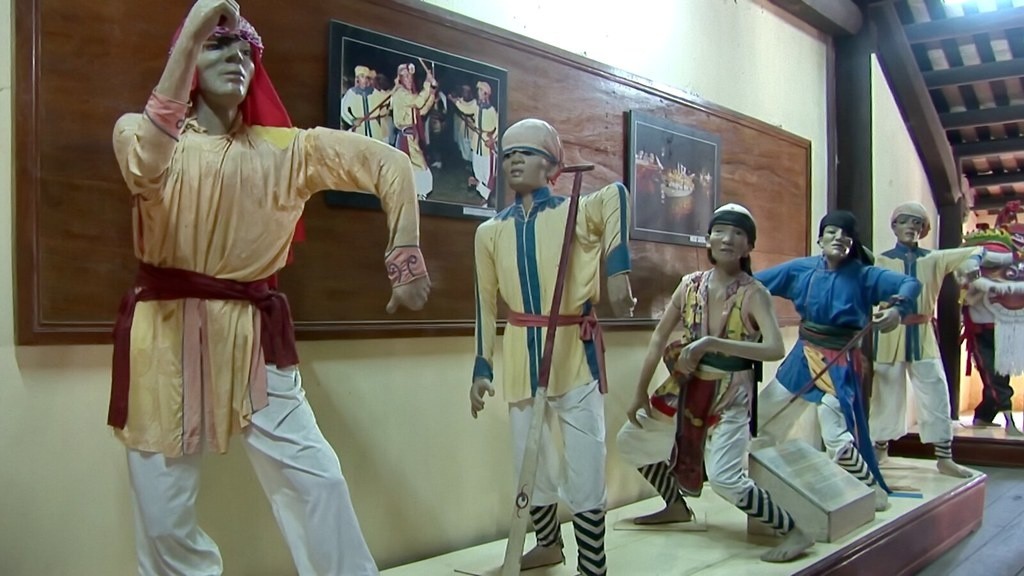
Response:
[107,0,433,576]
[868,202,988,478]
[616,203,816,563]
[470,118,638,576]
[341,56,497,209]
[749,211,924,512]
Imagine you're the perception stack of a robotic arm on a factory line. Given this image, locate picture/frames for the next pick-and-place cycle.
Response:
[321,18,507,223]
[629,106,722,247]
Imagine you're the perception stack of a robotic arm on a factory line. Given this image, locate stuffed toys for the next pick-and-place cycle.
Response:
[957,200,1024,435]
